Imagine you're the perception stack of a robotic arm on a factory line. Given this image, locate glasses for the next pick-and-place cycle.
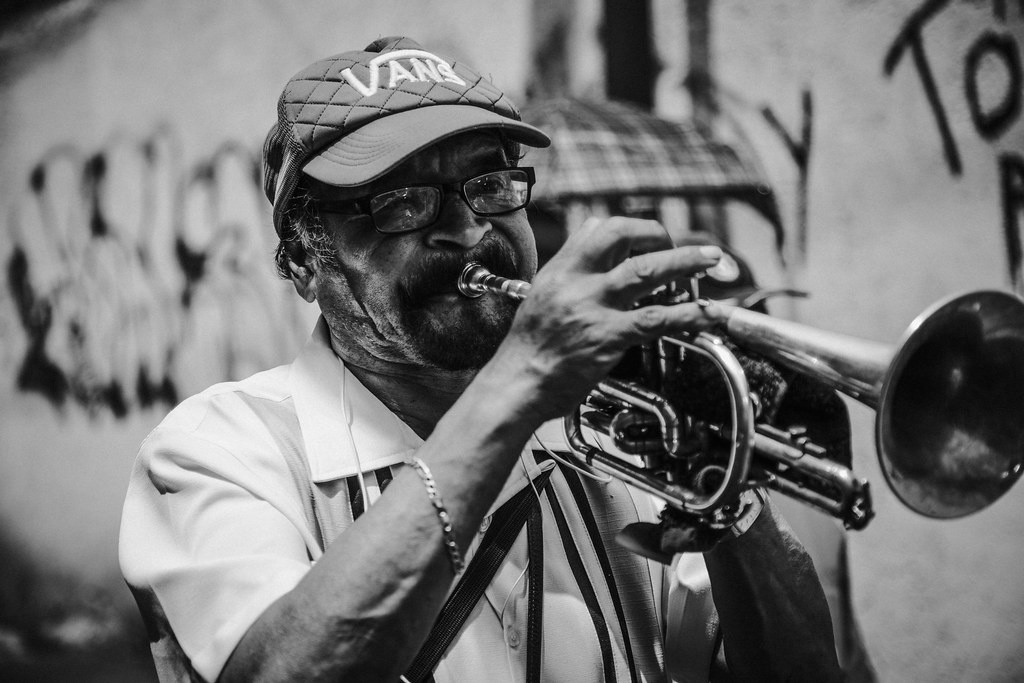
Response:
[289,166,537,237]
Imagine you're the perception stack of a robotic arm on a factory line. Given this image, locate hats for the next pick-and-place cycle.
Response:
[261,34,553,247]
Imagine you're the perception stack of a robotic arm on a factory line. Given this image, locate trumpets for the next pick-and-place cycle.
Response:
[457,262,1024,532]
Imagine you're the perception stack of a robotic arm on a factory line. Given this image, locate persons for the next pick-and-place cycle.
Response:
[114,33,840,683]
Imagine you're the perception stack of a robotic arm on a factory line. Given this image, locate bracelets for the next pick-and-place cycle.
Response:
[403,454,467,576]
[699,487,771,543]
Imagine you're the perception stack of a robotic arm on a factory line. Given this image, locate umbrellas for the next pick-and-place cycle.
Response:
[509,87,786,255]
